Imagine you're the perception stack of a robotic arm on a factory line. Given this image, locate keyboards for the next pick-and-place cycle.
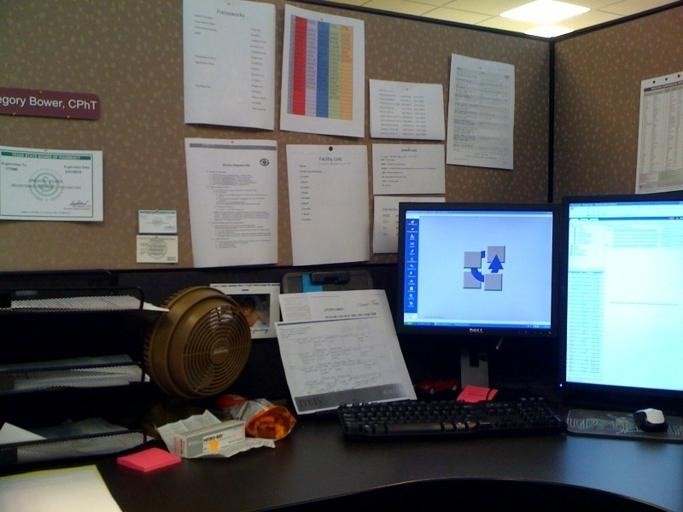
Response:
[336,401,568,445]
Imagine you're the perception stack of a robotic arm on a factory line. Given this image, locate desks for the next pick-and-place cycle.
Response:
[0,395,683,512]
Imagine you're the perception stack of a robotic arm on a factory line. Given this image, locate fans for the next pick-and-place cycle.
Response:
[144,285,252,433]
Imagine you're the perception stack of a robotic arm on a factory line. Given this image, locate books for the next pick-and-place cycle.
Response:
[12,358,149,392]
[1,465,124,512]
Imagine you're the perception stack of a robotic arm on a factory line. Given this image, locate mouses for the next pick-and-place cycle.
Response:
[632,407,669,434]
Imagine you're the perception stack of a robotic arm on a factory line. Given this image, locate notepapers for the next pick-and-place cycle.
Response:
[116,447,181,473]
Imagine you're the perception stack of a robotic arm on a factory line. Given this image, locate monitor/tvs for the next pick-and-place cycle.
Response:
[558,190,683,405]
[393,202,559,401]
